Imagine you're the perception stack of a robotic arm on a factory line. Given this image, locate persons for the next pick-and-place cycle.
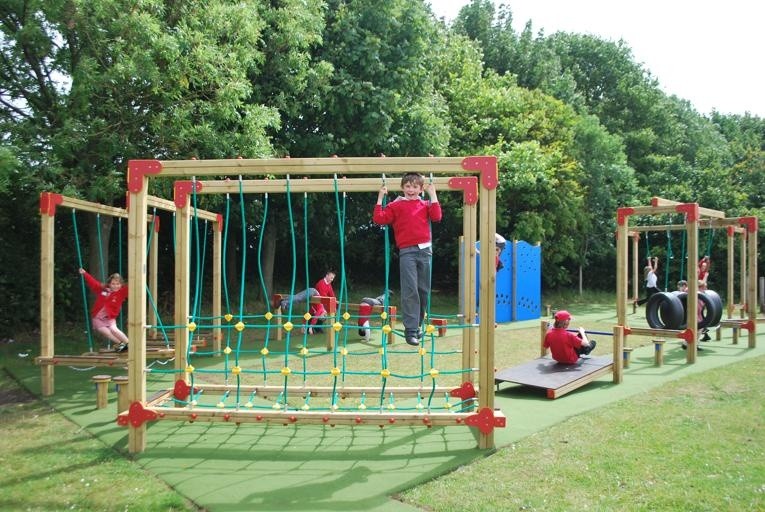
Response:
[677,279,689,293]
[356,289,394,343]
[632,255,663,308]
[372,171,443,347]
[78,265,129,353]
[315,272,337,334]
[280,287,328,336]
[680,272,710,352]
[700,328,710,342]
[542,309,597,365]
[698,254,712,282]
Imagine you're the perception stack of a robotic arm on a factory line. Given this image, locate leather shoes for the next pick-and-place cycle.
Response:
[404,327,422,345]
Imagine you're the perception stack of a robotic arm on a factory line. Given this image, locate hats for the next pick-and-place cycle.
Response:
[554,310,572,321]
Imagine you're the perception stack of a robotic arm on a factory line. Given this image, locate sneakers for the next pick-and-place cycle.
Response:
[113,342,129,352]
[585,339,596,355]
[280,298,288,311]
[383,288,395,297]
